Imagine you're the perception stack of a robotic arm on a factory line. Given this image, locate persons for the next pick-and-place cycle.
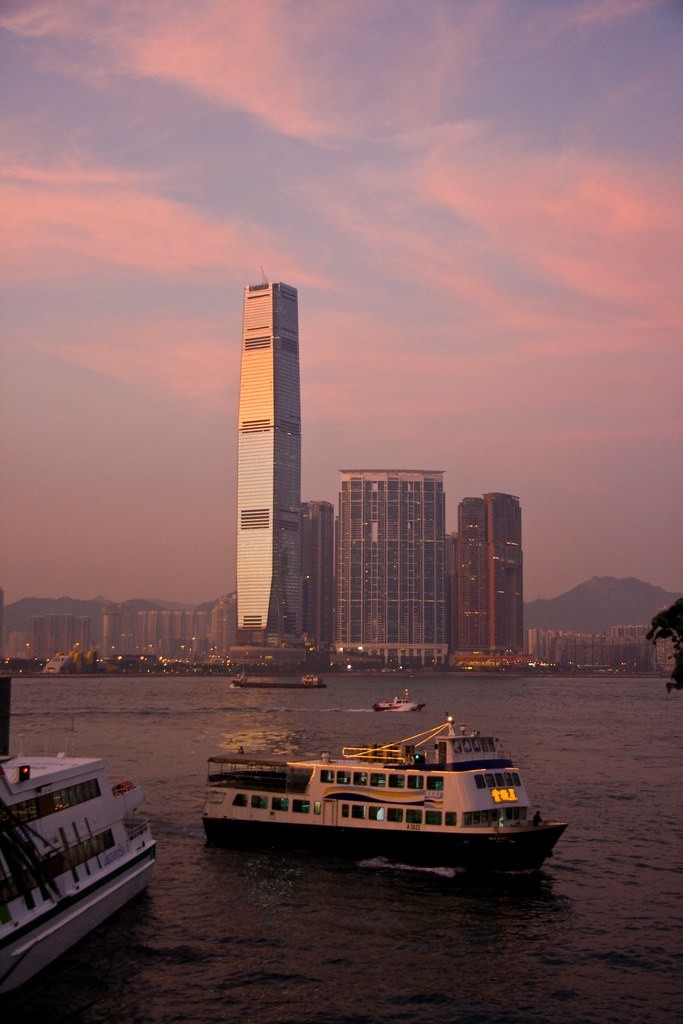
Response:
[405,753,414,765]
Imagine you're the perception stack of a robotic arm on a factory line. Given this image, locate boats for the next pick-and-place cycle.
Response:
[373,691,427,714]
[233,668,327,688]
[200,715,570,876]
[1,675,160,1024]
